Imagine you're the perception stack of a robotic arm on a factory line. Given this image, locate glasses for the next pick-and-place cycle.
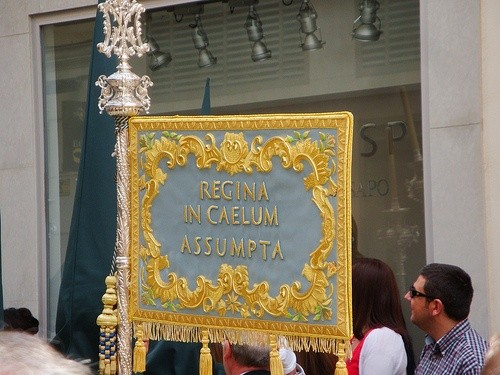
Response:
[410,287,425,299]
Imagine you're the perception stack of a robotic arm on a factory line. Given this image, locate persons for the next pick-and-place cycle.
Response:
[277,258,417,375]
[221,328,271,375]
[403,262,489,375]
